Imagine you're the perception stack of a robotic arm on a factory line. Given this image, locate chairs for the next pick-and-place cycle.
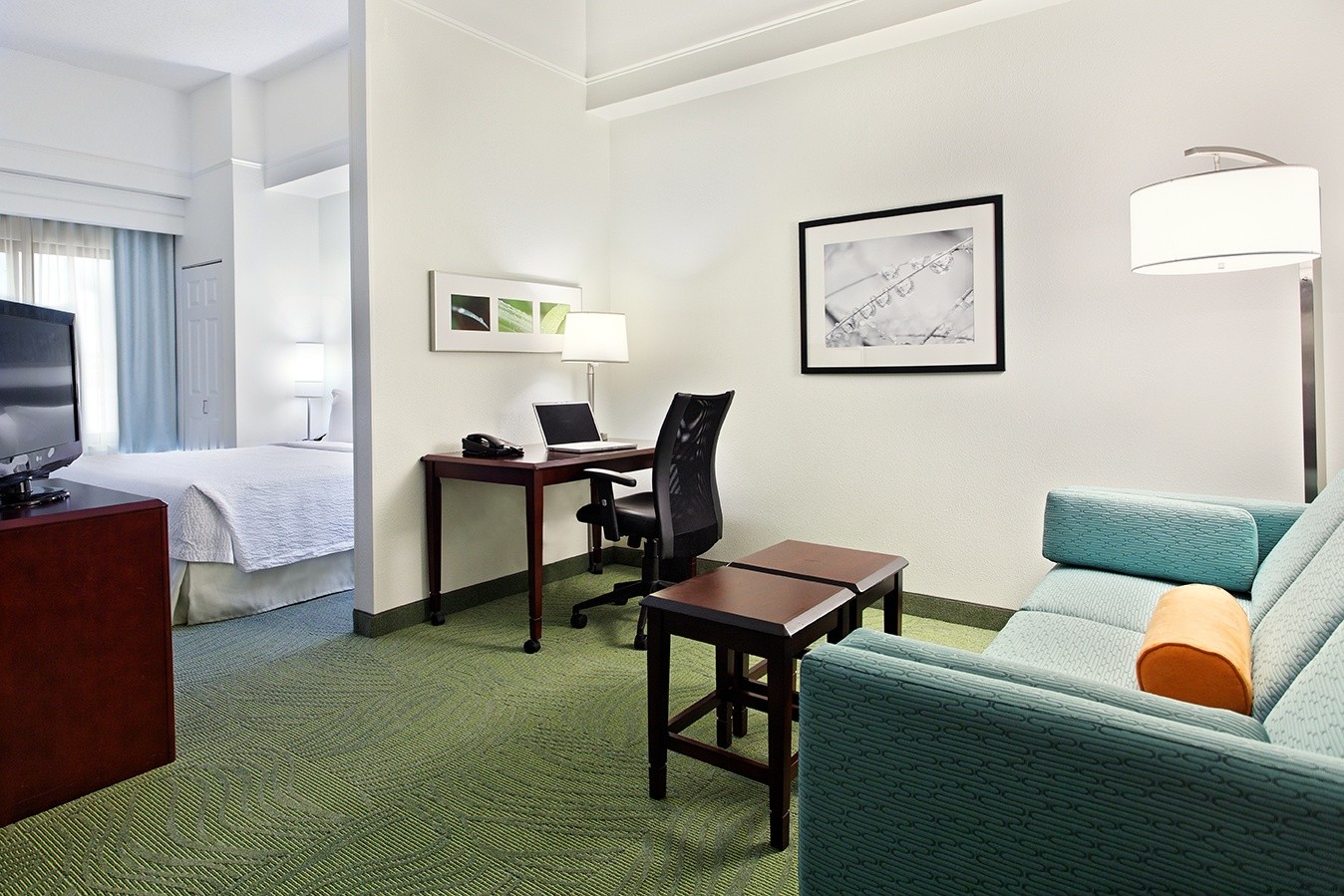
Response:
[573,390,736,650]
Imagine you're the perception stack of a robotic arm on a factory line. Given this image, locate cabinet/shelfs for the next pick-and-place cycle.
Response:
[0,481,176,828]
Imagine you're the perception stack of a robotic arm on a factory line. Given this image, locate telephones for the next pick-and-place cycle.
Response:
[461,433,524,459]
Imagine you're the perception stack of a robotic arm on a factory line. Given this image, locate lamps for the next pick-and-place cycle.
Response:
[1129,146,1324,505]
[291,343,328,438]
[560,311,629,445]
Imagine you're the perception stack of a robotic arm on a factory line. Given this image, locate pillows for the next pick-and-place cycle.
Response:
[840,626,1270,741]
[1042,485,1260,593]
[329,388,354,443]
[1135,578,1255,715]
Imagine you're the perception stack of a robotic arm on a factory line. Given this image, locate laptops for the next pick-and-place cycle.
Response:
[531,401,638,453]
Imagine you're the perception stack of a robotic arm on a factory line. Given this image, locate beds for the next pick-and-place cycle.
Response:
[49,438,355,624]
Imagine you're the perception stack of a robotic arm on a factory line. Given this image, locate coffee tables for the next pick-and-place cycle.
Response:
[636,565,855,851]
[729,539,909,743]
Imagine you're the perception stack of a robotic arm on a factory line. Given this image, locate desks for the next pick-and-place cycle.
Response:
[421,439,657,652]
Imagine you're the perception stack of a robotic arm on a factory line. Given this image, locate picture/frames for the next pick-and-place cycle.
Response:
[799,194,1005,377]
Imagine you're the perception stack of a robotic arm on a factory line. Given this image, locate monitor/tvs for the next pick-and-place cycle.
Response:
[0,299,83,509]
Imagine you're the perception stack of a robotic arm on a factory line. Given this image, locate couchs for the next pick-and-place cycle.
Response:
[787,472,1344,896]
